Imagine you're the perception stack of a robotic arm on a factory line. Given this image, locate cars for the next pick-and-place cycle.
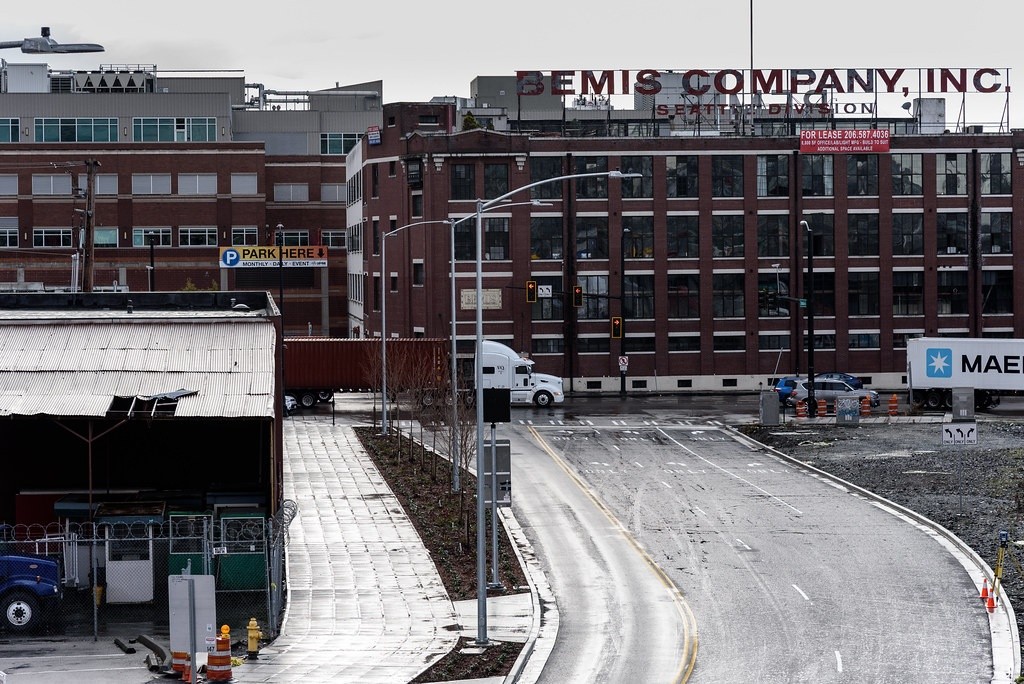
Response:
[814,371,863,390]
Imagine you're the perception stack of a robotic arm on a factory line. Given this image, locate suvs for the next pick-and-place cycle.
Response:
[785,378,881,414]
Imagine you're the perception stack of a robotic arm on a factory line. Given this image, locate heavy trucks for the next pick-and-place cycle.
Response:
[279,338,565,407]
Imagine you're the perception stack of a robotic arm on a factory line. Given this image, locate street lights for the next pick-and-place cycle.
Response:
[452,202,555,492]
[380,219,455,435]
[475,170,645,643]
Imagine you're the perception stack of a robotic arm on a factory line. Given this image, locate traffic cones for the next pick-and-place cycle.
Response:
[246,618,263,660]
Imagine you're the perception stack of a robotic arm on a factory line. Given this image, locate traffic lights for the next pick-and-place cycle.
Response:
[573,286,582,307]
[611,316,622,338]
[526,280,537,302]
